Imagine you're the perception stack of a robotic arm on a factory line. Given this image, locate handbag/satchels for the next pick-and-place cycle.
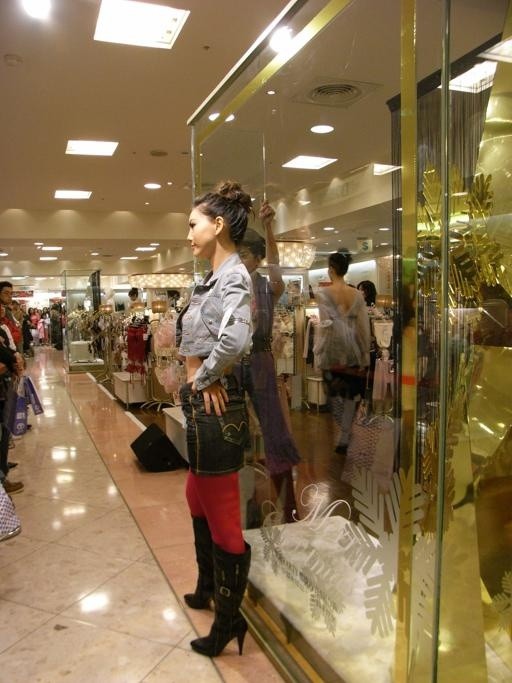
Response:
[10,392,28,436]
[24,376,45,416]
[371,358,396,402]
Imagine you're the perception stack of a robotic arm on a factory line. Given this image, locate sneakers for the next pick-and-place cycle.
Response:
[1,479,25,496]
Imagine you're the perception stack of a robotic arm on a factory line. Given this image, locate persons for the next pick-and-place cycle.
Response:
[313,246,373,455]
[0,277,61,510]
[127,287,145,310]
[236,198,305,532]
[356,280,377,306]
[174,177,256,659]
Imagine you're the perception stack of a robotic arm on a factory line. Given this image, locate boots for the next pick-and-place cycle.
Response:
[189,538,253,659]
[183,514,218,611]
